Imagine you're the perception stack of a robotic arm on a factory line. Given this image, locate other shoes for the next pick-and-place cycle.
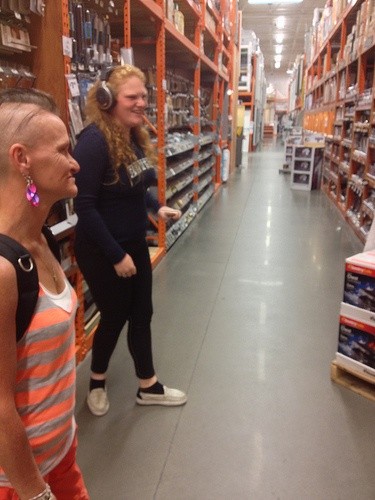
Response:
[136,383,188,406]
[87,381,109,416]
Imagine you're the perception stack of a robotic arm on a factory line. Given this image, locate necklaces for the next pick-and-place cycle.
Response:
[0,213,58,283]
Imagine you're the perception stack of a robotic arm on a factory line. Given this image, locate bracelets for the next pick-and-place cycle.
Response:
[28,483,52,500]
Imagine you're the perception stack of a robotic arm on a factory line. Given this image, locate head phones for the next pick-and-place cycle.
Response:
[95,65,118,111]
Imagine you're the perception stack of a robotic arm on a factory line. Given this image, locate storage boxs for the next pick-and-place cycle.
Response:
[339,248,375,327]
[335,315,375,381]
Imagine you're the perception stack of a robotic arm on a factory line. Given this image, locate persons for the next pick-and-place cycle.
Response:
[71,65,187,417]
[0,86,91,500]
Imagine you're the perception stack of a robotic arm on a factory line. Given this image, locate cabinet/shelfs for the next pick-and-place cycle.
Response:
[0,0,375,369]
